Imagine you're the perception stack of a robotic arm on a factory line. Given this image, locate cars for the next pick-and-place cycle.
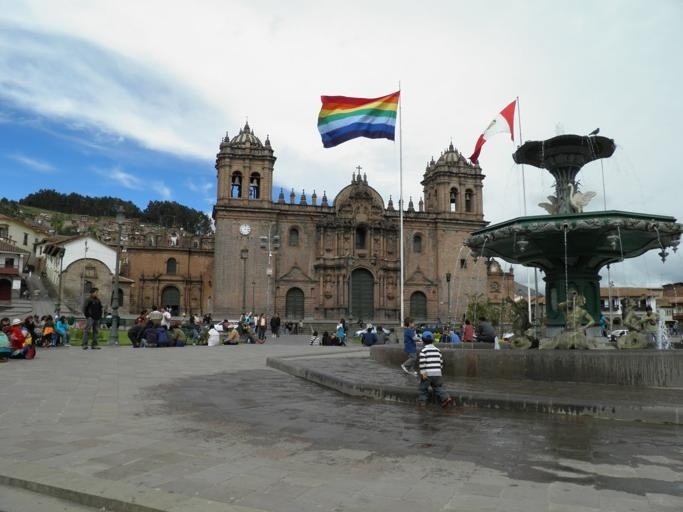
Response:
[207,319,239,330]
[609,329,628,343]
[502,333,514,340]
[351,325,390,337]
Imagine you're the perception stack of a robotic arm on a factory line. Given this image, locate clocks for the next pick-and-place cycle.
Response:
[239,223,252,236]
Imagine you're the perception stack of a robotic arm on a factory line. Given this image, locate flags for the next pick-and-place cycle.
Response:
[468,99,515,165]
[318,89,400,148]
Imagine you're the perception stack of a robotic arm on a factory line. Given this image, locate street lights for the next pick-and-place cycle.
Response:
[107,220,124,347]
[251,279,256,313]
[258,235,281,333]
[240,243,249,318]
[55,245,67,317]
[439,269,452,320]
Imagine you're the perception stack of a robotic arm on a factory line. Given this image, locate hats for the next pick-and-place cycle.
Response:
[421,331,434,340]
[11,318,23,326]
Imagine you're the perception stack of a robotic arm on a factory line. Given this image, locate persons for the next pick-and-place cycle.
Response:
[285,320,304,335]
[0,315,71,362]
[128,306,187,348]
[642,306,661,345]
[419,317,497,343]
[310,319,348,346]
[271,313,280,338]
[400,317,418,375]
[82,288,103,350]
[189,311,266,346]
[617,297,647,348]
[418,331,451,406]
[506,290,596,349]
[357,319,395,346]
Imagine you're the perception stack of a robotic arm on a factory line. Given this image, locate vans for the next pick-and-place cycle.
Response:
[414,321,464,335]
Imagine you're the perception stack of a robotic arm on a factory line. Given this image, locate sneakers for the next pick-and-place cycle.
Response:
[401,364,419,377]
[82,344,89,349]
[92,344,102,349]
[440,397,452,408]
[415,401,426,407]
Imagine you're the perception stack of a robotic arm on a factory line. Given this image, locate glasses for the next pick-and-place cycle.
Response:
[3,323,11,327]
